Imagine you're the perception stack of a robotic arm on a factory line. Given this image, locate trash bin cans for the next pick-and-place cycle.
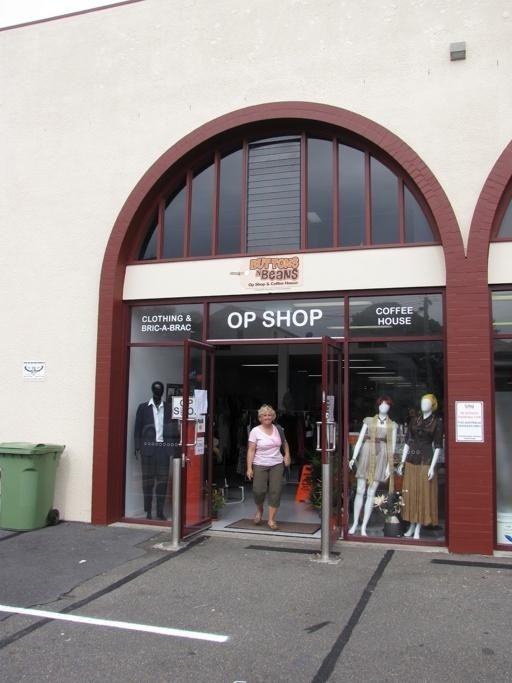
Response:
[0,442,66,531]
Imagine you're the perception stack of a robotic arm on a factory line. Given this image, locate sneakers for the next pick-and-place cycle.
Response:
[254,513,263,525]
[267,521,279,530]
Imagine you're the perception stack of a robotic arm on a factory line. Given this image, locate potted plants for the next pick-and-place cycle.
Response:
[309,457,341,542]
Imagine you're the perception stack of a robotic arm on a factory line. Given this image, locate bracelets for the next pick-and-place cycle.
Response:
[399,461,404,464]
[351,457,356,461]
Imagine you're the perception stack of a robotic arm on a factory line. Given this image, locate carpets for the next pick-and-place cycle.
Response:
[208,486,321,534]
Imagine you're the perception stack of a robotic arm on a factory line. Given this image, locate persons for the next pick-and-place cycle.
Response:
[133,380,180,522]
[347,393,399,538]
[395,393,443,538]
[246,403,293,530]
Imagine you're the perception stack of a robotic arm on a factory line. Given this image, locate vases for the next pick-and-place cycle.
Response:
[382,514,403,537]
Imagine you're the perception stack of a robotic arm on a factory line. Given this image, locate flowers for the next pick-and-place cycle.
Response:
[373,489,406,517]
